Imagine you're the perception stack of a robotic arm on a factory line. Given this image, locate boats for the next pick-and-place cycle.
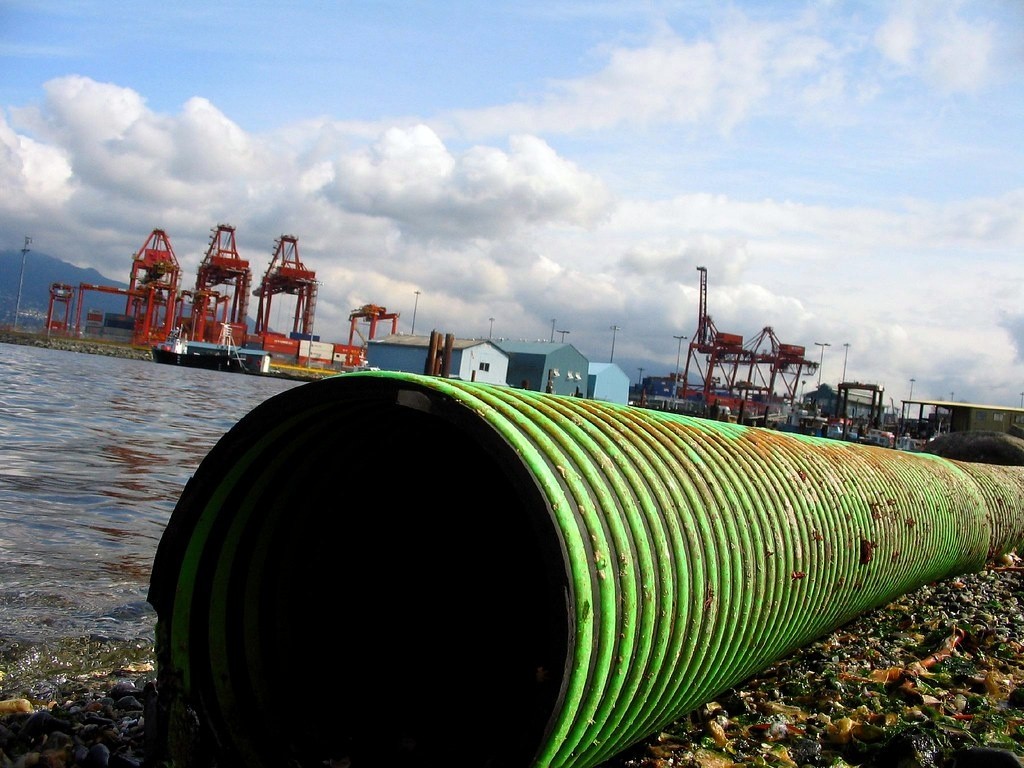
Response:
[150,323,325,382]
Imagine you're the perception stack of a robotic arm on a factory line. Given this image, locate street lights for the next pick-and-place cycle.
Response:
[814,343,831,404]
[841,343,851,385]
[489,317,495,340]
[410,291,421,335]
[906,379,916,425]
[549,318,556,342]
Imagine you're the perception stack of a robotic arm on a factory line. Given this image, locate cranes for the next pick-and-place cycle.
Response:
[680,266,821,423]
[125,228,181,352]
[188,223,251,350]
[253,236,318,340]
[340,304,399,372]
